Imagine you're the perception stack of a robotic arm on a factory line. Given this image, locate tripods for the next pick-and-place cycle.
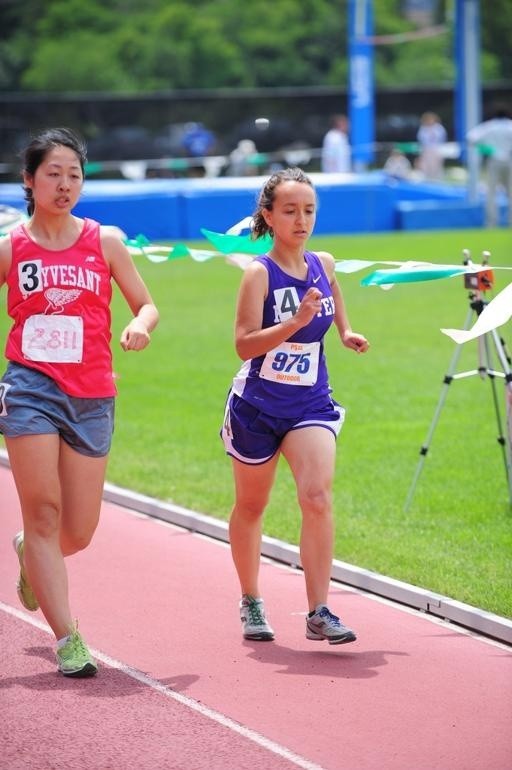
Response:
[404,292,512,517]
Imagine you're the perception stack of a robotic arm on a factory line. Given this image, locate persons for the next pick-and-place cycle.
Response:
[380,147,413,171]
[218,168,369,645]
[466,113,511,228]
[181,122,218,177]
[0,125,162,676]
[321,111,354,174]
[415,111,448,175]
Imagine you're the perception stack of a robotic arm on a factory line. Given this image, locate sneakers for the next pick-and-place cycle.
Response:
[13,529,39,610]
[305,604,357,645]
[55,619,98,678]
[239,593,277,641]
